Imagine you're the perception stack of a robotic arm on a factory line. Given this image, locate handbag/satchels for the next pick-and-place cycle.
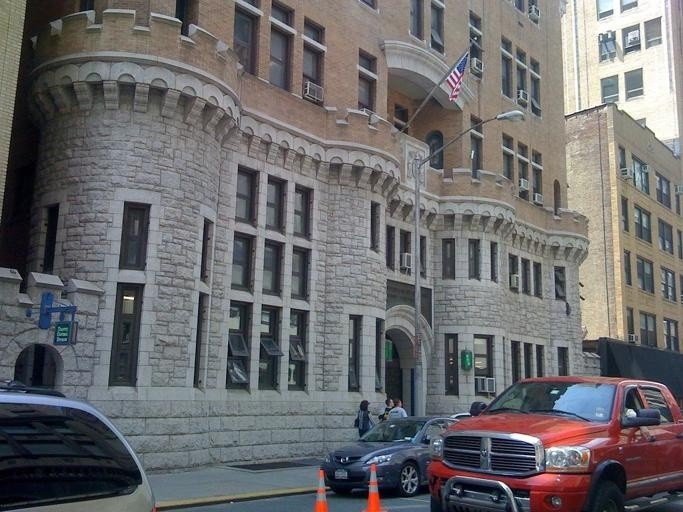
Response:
[354,413,360,427]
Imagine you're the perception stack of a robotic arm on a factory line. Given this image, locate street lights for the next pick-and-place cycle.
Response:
[413,111,527,416]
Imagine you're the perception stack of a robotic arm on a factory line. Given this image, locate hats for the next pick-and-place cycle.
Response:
[361,400,370,405]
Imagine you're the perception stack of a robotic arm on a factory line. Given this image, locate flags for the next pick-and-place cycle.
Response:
[445,52,468,102]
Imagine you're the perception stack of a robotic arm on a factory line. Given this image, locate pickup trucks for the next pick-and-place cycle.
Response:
[428,378,683,511]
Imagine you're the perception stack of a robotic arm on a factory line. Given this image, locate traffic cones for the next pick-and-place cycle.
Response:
[315,470,332,512]
[361,461,384,512]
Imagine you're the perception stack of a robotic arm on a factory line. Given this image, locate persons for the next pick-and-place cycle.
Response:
[355,397,407,438]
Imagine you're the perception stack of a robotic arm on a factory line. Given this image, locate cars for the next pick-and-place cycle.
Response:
[320,416,460,496]
[0,377,155,511]
